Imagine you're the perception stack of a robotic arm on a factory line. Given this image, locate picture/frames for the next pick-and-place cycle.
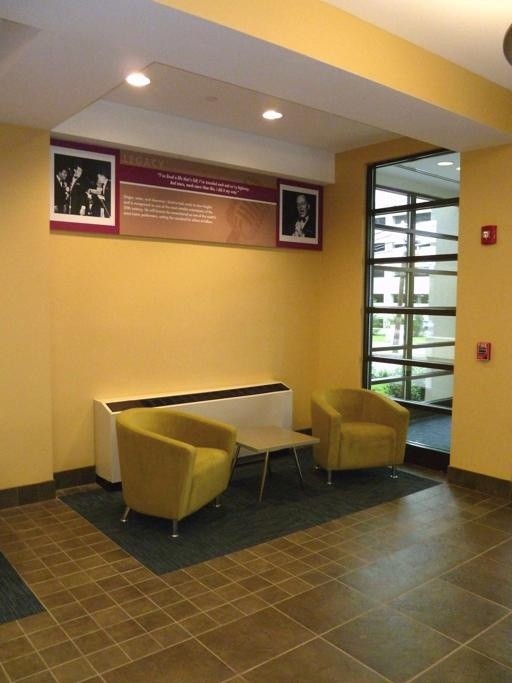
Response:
[276,177,324,252]
[50,137,120,232]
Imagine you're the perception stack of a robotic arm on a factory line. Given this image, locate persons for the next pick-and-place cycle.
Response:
[287,194,315,237]
[54,165,110,217]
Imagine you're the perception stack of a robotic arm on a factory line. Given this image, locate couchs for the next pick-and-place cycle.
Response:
[116,407,238,539]
[311,386,409,486]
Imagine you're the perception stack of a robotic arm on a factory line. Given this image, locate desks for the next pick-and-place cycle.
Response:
[229,426,321,505]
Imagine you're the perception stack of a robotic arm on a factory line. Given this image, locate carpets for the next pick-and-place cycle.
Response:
[0,553,46,627]
[58,444,443,577]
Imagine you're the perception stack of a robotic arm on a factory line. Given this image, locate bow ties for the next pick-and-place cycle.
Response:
[299,217,306,222]
